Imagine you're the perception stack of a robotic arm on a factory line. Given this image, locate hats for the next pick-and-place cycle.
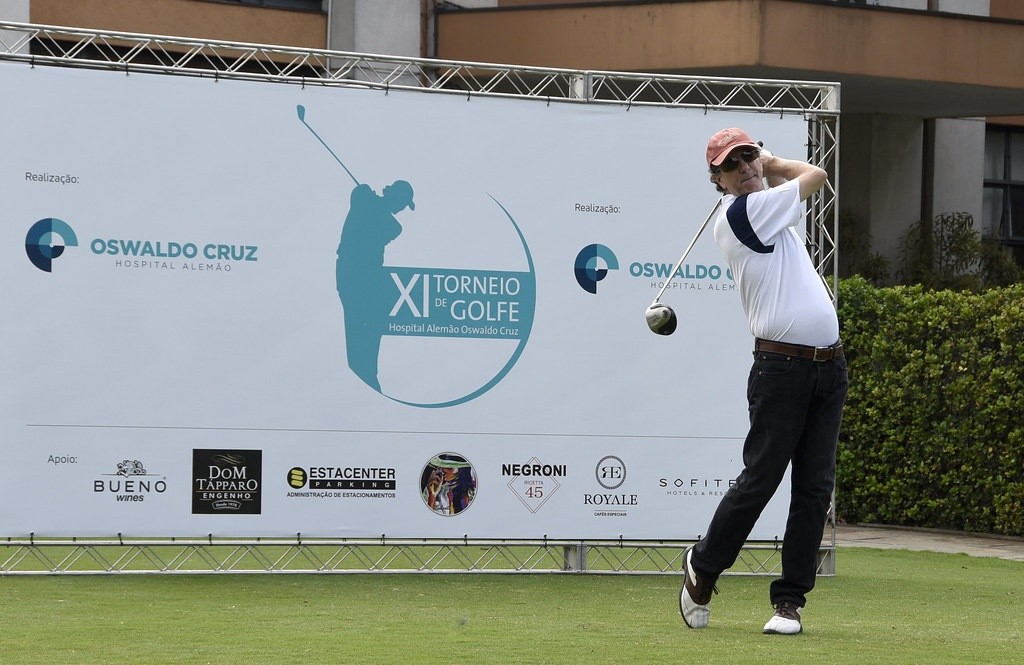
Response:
[706,127,758,172]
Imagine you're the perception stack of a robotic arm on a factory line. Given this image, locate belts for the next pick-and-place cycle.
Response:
[758,340,843,363]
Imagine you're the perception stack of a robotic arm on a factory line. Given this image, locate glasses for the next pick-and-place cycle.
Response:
[714,148,759,174]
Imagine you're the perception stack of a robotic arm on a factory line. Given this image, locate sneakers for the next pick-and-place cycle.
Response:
[679,545,721,628]
[763,600,802,634]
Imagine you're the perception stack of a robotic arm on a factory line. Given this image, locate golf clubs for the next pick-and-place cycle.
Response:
[645,140,765,337]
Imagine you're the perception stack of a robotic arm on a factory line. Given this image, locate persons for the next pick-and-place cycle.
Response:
[679,127,848,634]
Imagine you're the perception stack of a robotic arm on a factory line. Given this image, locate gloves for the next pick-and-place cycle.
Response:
[754,141,773,158]
